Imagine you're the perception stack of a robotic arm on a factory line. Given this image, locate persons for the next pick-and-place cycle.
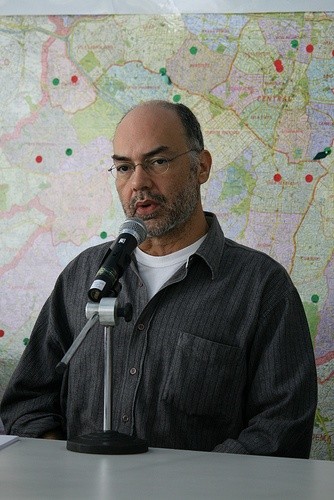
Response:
[0,100,319,459]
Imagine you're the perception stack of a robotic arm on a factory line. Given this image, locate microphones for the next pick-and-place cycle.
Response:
[87,217,147,302]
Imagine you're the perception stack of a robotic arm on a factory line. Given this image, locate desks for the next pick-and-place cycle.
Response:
[0,436,334,500]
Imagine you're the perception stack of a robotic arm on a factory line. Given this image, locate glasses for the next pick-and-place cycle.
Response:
[108,148,196,180]
